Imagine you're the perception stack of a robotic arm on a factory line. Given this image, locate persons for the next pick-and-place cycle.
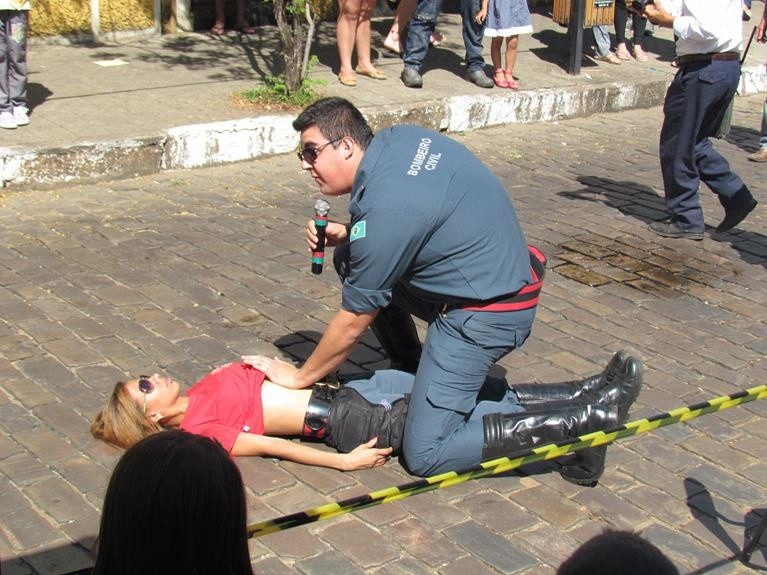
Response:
[240,95,627,490]
[89,349,643,473]
[335,0,388,88]
[382,0,445,59]
[748,0,767,162]
[60,431,257,575]
[208,0,258,35]
[553,528,681,575]
[741,0,754,21]
[0,0,36,131]
[401,0,495,90]
[612,0,652,62]
[620,0,757,243]
[472,0,534,90]
[565,24,619,64]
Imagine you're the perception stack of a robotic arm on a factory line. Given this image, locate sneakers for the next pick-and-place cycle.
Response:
[466,68,494,88]
[401,68,423,87]
[339,71,357,86]
[357,65,386,80]
[0,112,17,129]
[13,107,30,125]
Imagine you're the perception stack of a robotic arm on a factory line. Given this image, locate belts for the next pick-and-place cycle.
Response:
[445,244,547,312]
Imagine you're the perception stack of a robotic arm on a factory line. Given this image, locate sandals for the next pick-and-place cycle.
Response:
[492,68,508,88]
[504,70,519,89]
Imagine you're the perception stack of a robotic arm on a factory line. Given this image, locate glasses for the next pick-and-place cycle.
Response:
[297,138,342,161]
[138,375,154,415]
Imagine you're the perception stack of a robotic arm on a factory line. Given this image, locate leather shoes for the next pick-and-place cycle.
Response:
[715,194,758,232]
[648,222,704,240]
[747,147,767,162]
[300,375,337,440]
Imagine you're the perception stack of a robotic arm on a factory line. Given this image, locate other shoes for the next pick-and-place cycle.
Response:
[212,16,255,34]
[589,41,680,67]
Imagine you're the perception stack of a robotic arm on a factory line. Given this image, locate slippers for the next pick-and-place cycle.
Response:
[382,31,446,58]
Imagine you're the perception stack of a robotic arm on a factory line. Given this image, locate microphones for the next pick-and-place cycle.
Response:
[311,199,329,274]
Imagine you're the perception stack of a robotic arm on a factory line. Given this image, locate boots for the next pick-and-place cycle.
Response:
[483,403,618,485]
[512,350,630,400]
[368,307,422,376]
[525,355,643,425]
